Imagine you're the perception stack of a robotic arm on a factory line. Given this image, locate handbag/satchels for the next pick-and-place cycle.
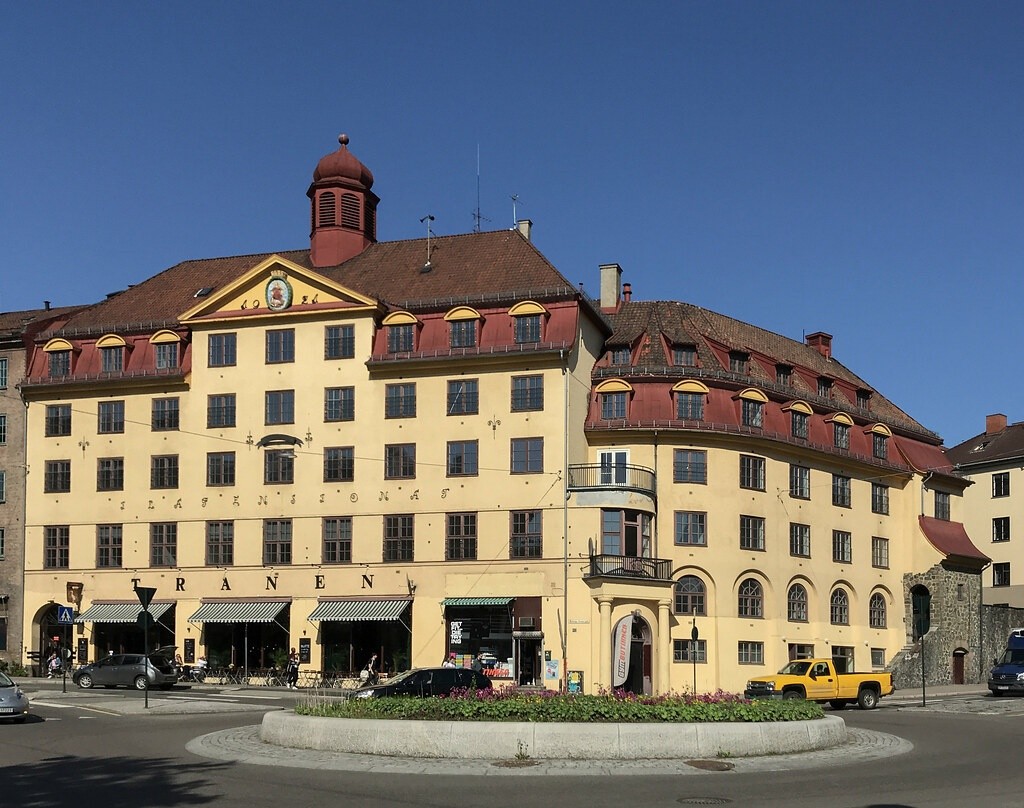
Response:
[360,670,369,681]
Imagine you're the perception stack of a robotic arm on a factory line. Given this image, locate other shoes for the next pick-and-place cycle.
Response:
[286,683,290,688]
[292,686,298,690]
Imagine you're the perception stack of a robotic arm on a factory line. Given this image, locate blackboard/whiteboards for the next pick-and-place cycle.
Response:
[299,638,310,663]
[78,638,88,666]
[184,639,195,663]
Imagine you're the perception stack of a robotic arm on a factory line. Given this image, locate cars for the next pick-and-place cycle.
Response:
[346,667,493,703]
[0,671,30,723]
[72,644,179,691]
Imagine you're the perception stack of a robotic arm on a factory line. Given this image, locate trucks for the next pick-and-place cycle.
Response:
[986,631,1024,699]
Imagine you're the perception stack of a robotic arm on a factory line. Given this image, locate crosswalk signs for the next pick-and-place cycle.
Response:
[58,606,74,624]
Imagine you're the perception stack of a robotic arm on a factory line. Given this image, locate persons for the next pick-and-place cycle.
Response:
[286,647,301,690]
[46,652,62,677]
[175,654,183,665]
[360,653,379,688]
[472,652,483,673]
[198,657,208,674]
[443,656,457,668]
[384,660,390,672]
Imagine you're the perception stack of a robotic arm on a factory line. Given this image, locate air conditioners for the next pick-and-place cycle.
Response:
[519,617,536,627]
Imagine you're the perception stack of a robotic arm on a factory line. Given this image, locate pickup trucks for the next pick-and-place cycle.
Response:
[744,658,896,711]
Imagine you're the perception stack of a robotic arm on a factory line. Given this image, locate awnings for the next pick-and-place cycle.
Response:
[439,598,514,625]
[187,602,290,634]
[513,630,542,640]
[306,600,412,633]
[73,604,175,634]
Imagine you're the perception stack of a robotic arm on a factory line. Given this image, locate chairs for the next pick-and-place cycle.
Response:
[178,666,286,686]
